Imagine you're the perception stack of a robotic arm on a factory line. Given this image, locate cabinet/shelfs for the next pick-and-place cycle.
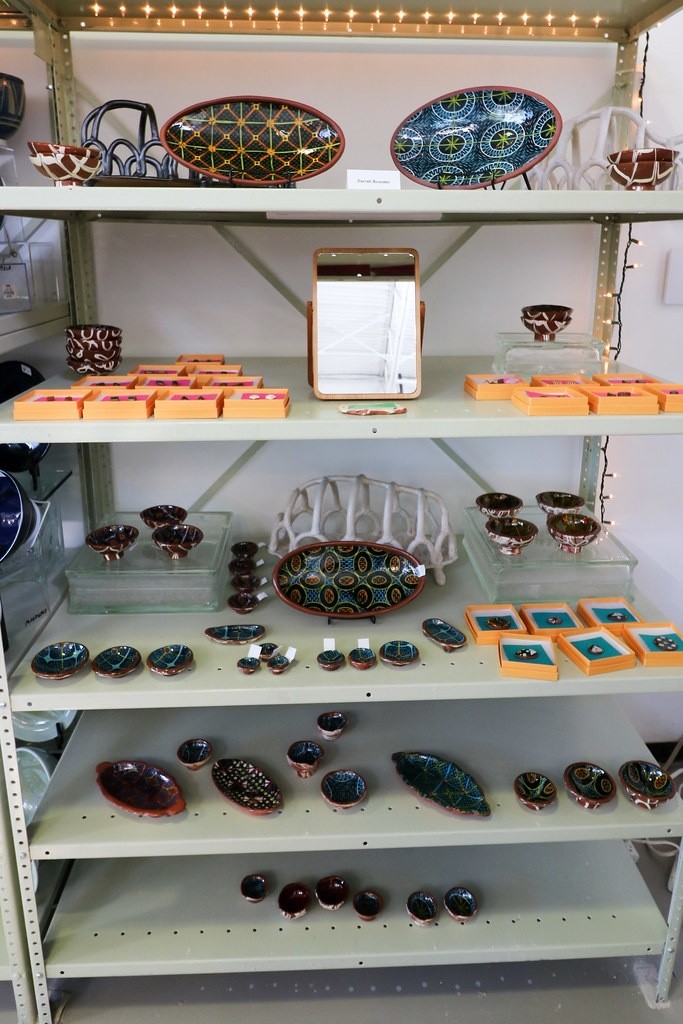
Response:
[0,0,683,1024]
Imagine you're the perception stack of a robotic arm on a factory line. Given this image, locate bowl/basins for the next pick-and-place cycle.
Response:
[514,772,557,810]
[476,492,524,521]
[286,740,326,777]
[236,640,420,674]
[563,761,617,809]
[241,872,479,925]
[485,518,539,555]
[0,468,41,562]
[85,525,139,562]
[320,769,368,808]
[176,738,213,770]
[536,492,585,519]
[316,711,347,740]
[547,513,601,554]
[140,505,187,530]
[619,760,677,809]
[606,148,680,191]
[27,141,102,186]
[520,304,573,340]
[63,324,123,375]
[152,524,204,560]
[227,542,259,614]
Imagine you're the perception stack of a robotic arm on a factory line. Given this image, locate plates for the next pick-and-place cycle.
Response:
[273,541,426,618]
[31,641,194,680]
[202,624,266,645]
[391,751,491,818]
[160,95,345,185]
[390,86,562,191]
[422,618,466,653]
[95,759,186,818]
[0,360,54,473]
[212,758,282,816]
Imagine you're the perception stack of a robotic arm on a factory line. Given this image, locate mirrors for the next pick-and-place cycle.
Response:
[305,248,427,401]
